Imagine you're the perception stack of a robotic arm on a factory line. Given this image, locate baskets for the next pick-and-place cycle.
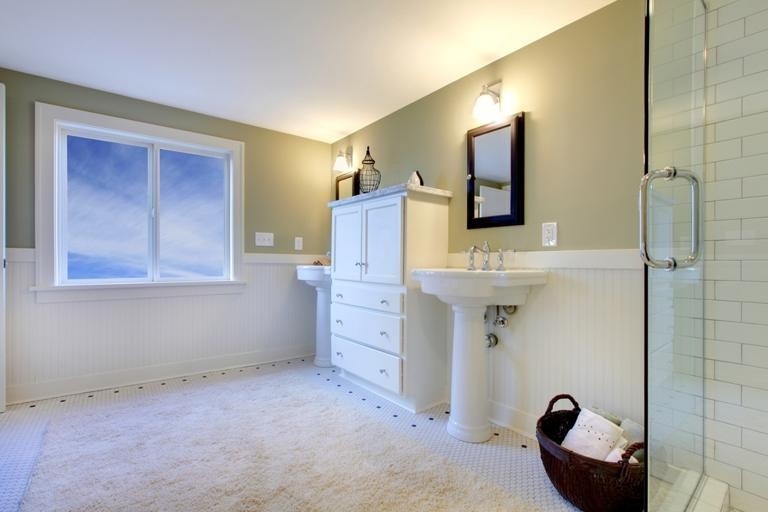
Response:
[536,393,644,512]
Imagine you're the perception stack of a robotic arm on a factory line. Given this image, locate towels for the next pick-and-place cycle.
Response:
[561,408,623,461]
[620,419,644,457]
[604,447,638,464]
[614,438,628,449]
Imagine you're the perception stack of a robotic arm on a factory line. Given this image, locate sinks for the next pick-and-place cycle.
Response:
[297,265,331,288]
[409,266,548,308]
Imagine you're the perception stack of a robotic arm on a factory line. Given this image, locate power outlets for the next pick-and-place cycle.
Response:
[542,223,557,245]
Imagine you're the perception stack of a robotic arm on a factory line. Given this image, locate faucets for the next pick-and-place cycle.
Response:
[471,240,490,270]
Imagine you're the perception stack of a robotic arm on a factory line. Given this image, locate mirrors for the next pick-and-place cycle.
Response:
[467,112,525,228]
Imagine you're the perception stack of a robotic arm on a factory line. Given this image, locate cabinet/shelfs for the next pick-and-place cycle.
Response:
[328,184,452,413]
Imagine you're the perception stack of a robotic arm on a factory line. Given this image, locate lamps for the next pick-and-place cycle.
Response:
[332,150,352,172]
[473,80,502,122]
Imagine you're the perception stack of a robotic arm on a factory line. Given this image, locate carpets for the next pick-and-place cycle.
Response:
[19,386,547,512]
